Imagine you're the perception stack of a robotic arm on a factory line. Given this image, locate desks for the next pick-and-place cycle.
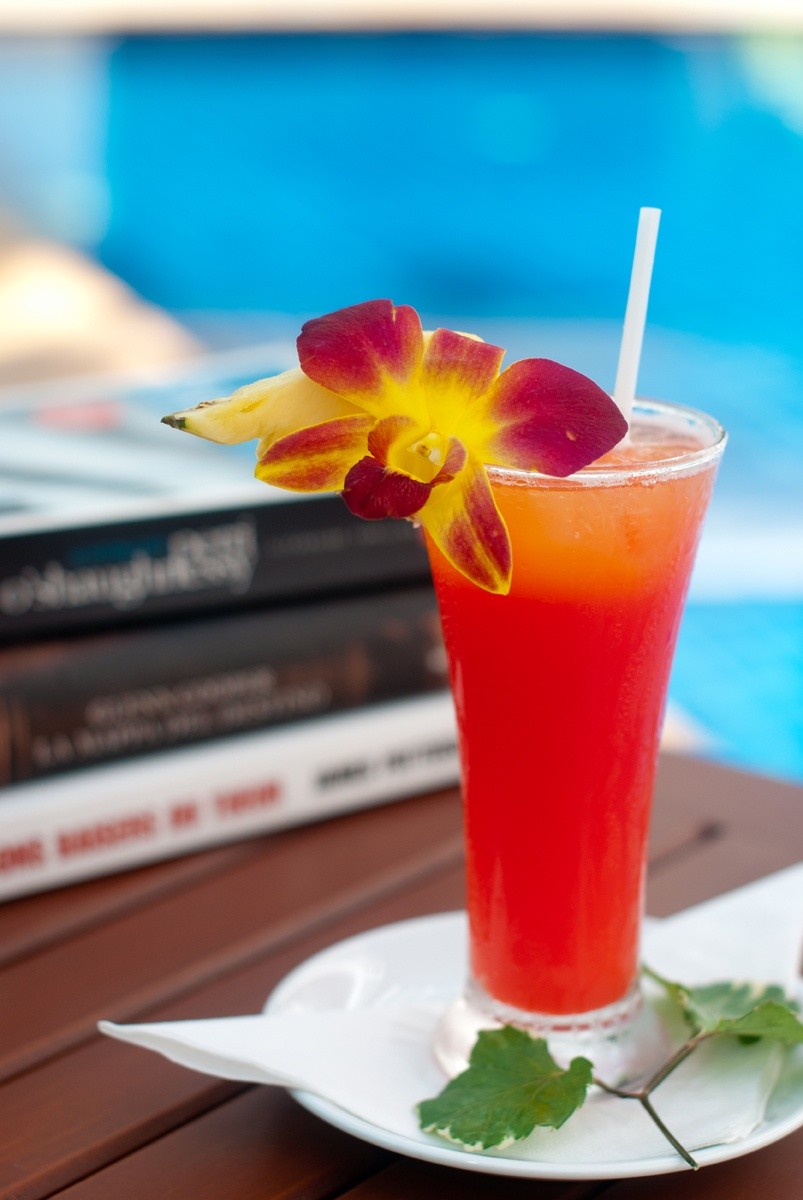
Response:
[5,753,800,1197]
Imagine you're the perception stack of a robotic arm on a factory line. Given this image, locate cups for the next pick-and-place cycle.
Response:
[429,395,731,1048]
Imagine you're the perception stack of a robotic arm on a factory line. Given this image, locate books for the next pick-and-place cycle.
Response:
[1,344,459,899]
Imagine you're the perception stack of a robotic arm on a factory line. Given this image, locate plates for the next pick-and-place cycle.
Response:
[260,896,803,1186]
